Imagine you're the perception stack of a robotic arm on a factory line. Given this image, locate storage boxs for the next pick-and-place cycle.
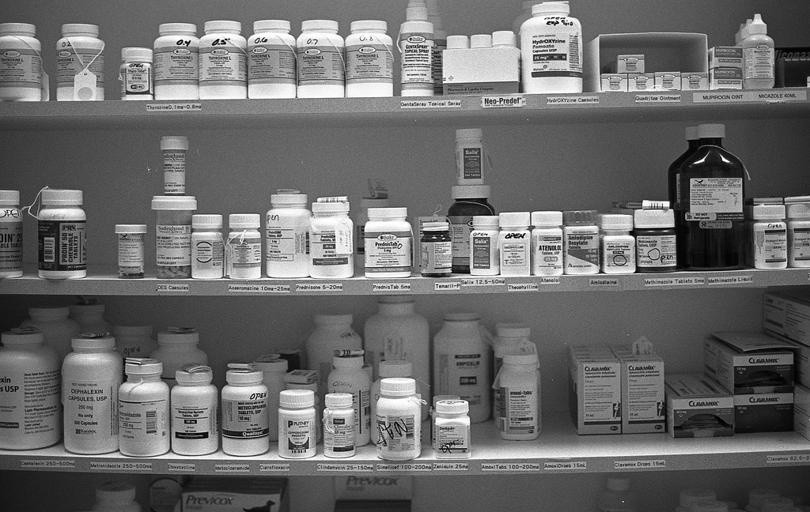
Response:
[568,346,665,436]
[704,290,809,439]
[665,372,734,438]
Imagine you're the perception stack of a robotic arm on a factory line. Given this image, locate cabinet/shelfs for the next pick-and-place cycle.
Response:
[1,84,809,475]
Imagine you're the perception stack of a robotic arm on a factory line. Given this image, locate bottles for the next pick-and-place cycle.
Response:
[736,12,776,90]
[151,195,197,279]
[114,224,148,279]
[277,387,318,459]
[0,188,88,279]
[3,21,105,103]
[119,20,394,100]
[377,376,423,461]
[158,135,188,196]
[422,220,453,277]
[2,300,541,449]
[448,184,497,274]
[753,195,808,270]
[118,356,170,456]
[395,1,586,98]
[261,187,355,280]
[324,391,355,458]
[666,120,755,270]
[90,484,140,510]
[190,213,262,280]
[433,400,471,459]
[467,200,678,276]
[165,364,220,456]
[456,127,487,185]
[364,207,417,280]
[221,363,267,457]
[62,326,124,456]
